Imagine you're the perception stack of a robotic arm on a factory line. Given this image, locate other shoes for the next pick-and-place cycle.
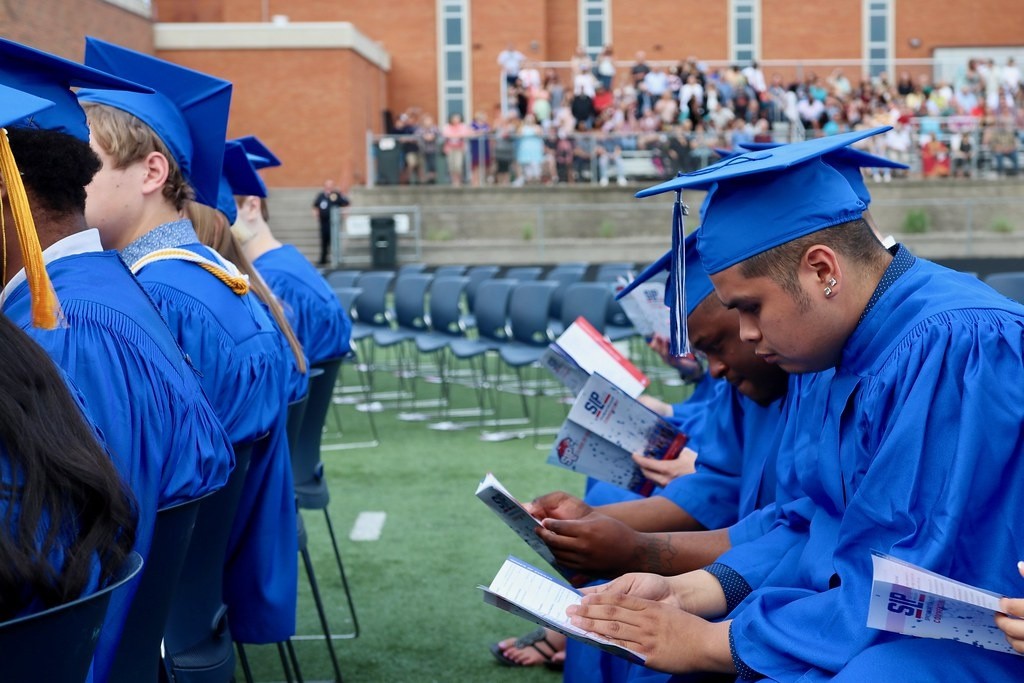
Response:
[599,177,610,189]
[616,176,629,188]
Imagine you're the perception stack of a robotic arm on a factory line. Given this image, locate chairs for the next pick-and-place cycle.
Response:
[985,272,1024,305]
[0,260,687,683]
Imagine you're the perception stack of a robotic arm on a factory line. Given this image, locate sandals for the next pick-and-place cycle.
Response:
[490,625,564,670]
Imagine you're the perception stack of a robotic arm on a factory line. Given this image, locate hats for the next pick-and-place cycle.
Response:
[614,225,715,320]
[633,126,894,358]
[0,84,72,331]
[737,142,911,212]
[228,135,283,170]
[0,38,156,143]
[215,141,270,225]
[714,148,744,161]
[73,34,233,210]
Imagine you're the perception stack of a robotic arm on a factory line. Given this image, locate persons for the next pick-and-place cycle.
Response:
[490,127,1023,681]
[391,40,1024,188]
[0,33,352,683]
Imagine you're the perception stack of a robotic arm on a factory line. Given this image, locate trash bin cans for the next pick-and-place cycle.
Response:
[370,215,396,270]
